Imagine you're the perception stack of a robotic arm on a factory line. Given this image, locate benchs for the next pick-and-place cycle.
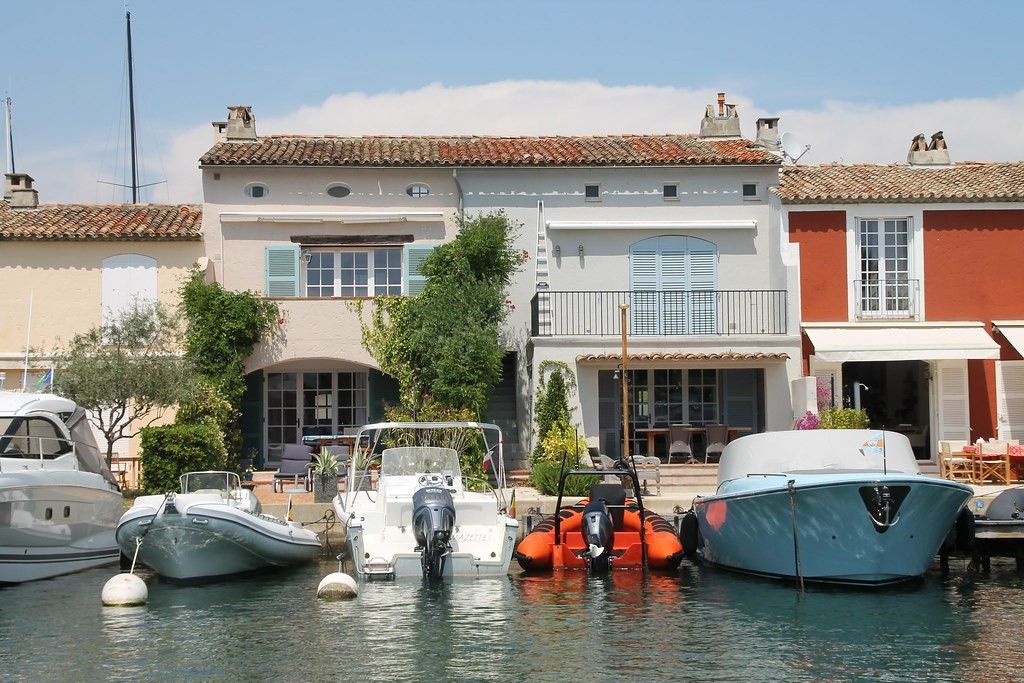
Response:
[588,446,662,497]
[100,453,128,490]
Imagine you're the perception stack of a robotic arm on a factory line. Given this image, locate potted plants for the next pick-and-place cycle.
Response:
[238,481,258,491]
[305,447,382,503]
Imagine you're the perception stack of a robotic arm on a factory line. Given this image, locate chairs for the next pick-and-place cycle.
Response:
[343,428,369,447]
[938,439,1024,486]
[665,423,729,465]
[905,425,930,456]
[273,443,350,493]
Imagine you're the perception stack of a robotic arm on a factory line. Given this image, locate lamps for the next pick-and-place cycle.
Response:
[859,383,869,391]
[301,248,312,265]
[579,245,584,256]
[923,367,933,381]
[612,370,620,380]
[556,245,560,257]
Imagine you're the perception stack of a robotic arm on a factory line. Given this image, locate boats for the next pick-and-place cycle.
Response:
[0,388,126,583]
[964,484,1024,554]
[515,463,685,575]
[115,470,323,579]
[688,427,975,589]
[331,421,519,580]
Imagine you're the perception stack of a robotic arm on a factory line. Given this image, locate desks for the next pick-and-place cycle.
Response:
[635,427,752,464]
[302,434,370,458]
[937,451,1024,484]
[887,427,922,434]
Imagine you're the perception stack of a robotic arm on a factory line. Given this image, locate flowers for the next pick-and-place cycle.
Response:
[246,465,257,473]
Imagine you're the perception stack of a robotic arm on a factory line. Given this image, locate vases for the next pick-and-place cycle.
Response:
[245,473,252,481]
